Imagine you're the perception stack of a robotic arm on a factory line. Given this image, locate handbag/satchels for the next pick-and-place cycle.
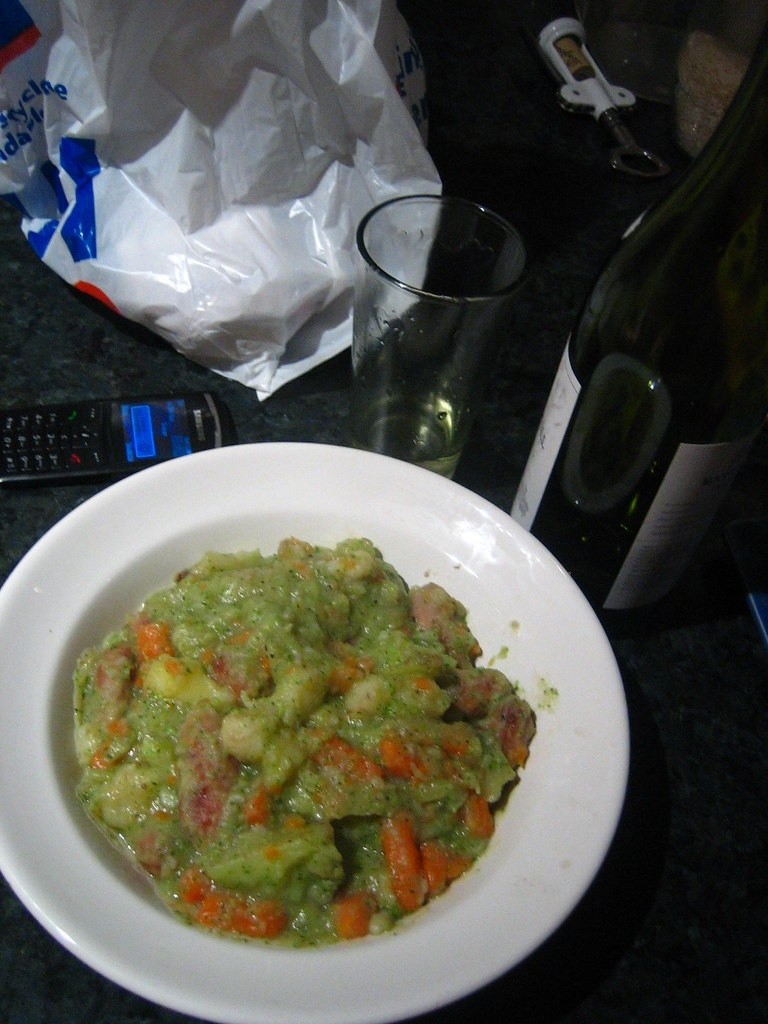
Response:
[0,0,447,404]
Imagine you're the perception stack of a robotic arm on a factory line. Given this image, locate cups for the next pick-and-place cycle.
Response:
[351,195,532,479]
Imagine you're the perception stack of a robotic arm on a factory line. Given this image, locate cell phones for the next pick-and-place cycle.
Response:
[0,390,239,487]
[721,515,768,652]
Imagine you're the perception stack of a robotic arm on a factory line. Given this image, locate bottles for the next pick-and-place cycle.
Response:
[510,21,768,640]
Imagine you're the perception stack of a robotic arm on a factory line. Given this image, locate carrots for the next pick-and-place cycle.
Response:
[91,621,492,938]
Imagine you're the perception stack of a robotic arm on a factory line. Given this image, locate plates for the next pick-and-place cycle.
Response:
[0,441,631,1024]
